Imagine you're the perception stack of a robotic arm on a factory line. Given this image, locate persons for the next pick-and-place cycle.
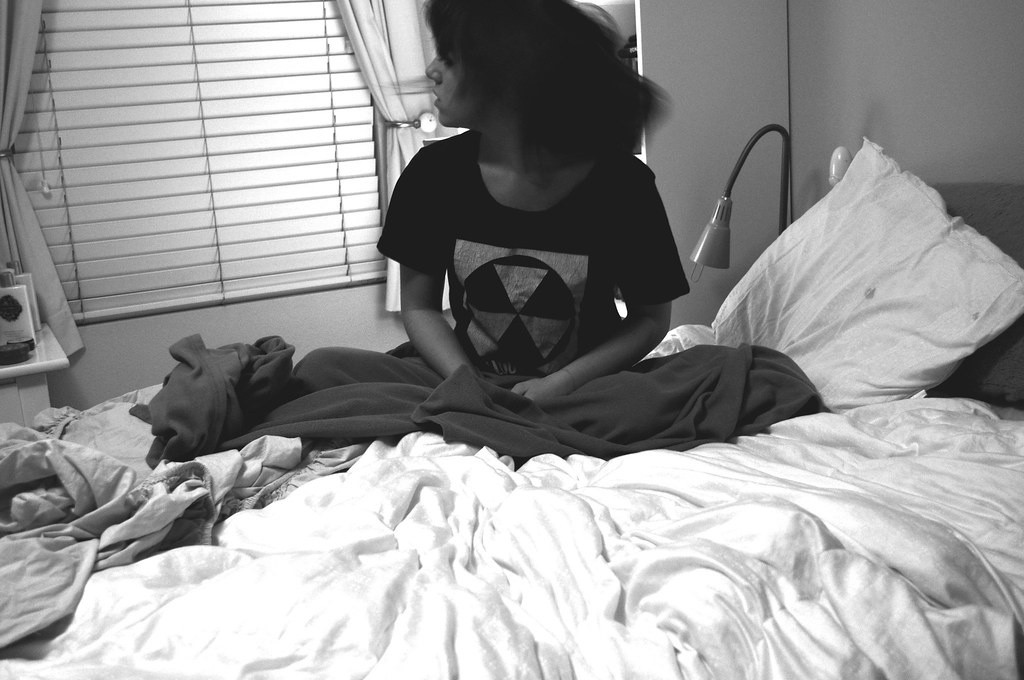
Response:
[287,0,810,420]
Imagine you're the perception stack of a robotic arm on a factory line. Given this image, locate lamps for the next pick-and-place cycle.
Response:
[689,124,791,289]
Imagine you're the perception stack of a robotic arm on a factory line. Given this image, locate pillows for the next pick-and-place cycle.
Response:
[712,137,1024,411]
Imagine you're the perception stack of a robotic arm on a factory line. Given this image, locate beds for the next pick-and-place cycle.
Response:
[0,186,1024,680]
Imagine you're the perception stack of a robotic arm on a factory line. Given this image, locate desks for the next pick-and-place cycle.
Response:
[0,325,69,427]
